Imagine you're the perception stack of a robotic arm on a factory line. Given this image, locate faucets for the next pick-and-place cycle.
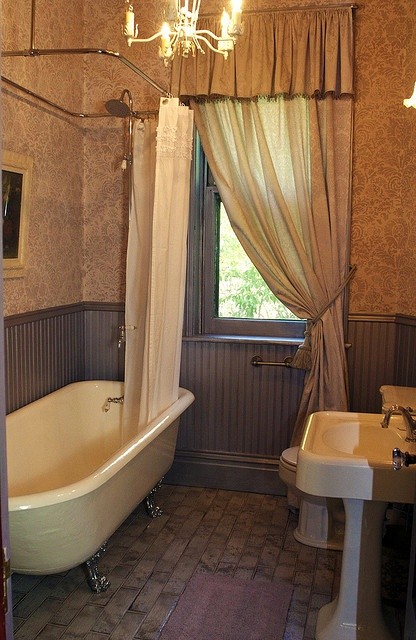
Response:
[378,403,416,444]
[117,324,137,349]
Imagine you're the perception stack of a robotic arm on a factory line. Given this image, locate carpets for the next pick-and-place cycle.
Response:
[157,572,294,640]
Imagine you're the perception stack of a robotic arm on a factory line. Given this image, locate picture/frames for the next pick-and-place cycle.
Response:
[0,148,34,280]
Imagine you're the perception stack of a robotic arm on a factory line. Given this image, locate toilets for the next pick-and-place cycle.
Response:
[278,385,416,550]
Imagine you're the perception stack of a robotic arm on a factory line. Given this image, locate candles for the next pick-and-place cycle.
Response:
[123,4,136,35]
[230,0,243,24]
[159,20,172,50]
[220,7,231,41]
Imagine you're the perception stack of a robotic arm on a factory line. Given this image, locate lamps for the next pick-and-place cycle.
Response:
[123,0,242,67]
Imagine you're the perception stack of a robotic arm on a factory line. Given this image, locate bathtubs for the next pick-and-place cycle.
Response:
[5,380,196,576]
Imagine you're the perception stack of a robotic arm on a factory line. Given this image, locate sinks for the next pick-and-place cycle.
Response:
[320,419,405,461]
[297,410,416,468]
